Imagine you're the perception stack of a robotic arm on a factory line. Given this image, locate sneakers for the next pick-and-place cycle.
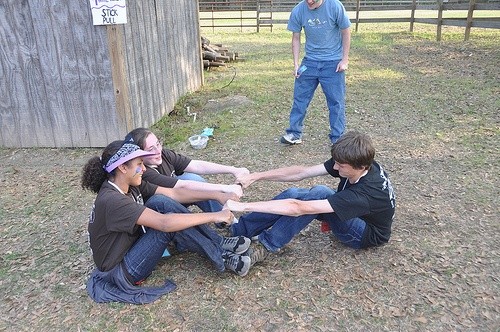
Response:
[222,236,250,277]
[215,228,229,237]
[243,242,267,267]
[281,133,302,145]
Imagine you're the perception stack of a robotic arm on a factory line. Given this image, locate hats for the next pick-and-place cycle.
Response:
[103,143,156,173]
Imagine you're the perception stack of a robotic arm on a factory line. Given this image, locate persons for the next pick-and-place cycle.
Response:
[80,139,252,286]
[124,128,259,240]
[280,0,352,144]
[222,131,396,267]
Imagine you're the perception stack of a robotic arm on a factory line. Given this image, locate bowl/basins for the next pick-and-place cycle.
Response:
[189,135,209,150]
